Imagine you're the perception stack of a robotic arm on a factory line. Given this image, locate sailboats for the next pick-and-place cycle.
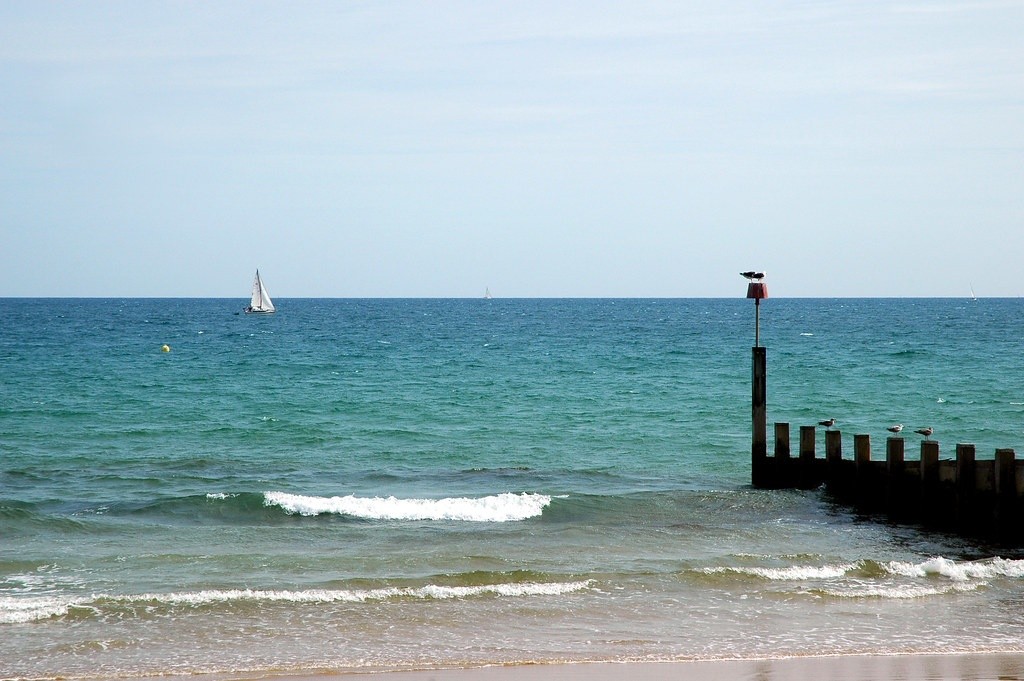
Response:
[244,269,275,315]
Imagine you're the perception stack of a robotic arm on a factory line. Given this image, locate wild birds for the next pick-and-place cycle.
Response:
[913,426,934,442]
[817,417,837,431]
[739,270,767,284]
[886,423,905,436]
